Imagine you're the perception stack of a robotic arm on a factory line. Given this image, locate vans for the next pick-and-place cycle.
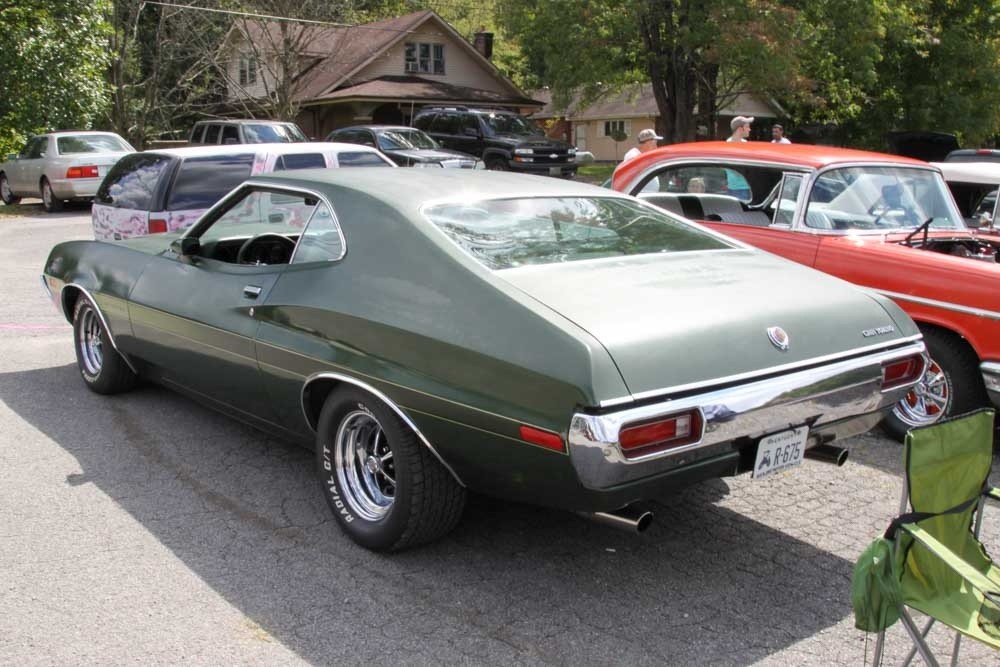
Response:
[186,118,313,150]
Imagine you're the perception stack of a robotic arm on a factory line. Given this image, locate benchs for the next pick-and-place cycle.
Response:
[644,193,753,221]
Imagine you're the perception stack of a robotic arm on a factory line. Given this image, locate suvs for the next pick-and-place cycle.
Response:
[411,104,579,182]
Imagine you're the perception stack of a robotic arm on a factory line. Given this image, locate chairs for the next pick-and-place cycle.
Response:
[780,207,836,231]
[705,209,771,224]
[851,407,1000,667]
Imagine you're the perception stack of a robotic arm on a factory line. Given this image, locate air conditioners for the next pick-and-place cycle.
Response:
[406,62,419,73]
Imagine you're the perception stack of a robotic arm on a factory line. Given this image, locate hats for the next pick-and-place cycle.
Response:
[639,129,664,141]
[730,117,754,130]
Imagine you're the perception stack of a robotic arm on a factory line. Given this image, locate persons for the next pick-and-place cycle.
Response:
[725,115,754,142]
[686,177,705,194]
[623,129,664,192]
[770,124,791,144]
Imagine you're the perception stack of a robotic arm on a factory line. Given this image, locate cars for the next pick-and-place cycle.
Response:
[542,141,1000,447]
[89,141,401,254]
[0,132,142,213]
[38,164,936,553]
[882,132,1000,237]
[322,124,487,173]
